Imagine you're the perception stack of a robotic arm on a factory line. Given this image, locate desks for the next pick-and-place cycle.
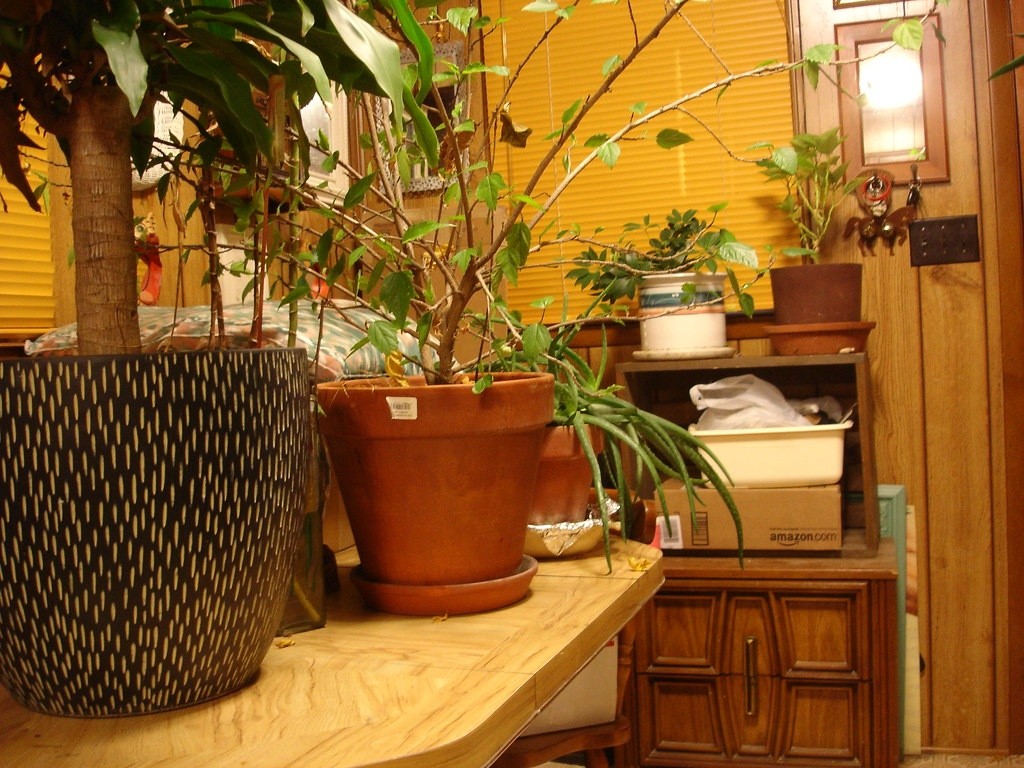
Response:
[0,538,668,768]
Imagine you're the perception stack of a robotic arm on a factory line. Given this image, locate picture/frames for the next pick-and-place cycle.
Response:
[372,44,469,199]
[832,14,951,188]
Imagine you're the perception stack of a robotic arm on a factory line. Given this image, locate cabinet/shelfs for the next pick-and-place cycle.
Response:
[640,536,899,768]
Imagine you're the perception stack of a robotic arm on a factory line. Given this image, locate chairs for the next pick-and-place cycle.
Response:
[492,615,636,768]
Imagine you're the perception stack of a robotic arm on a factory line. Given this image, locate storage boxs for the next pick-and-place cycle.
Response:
[654,477,844,552]
[686,420,852,488]
[517,636,619,737]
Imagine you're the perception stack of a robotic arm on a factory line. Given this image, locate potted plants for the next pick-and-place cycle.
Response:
[0,0,958,722]
